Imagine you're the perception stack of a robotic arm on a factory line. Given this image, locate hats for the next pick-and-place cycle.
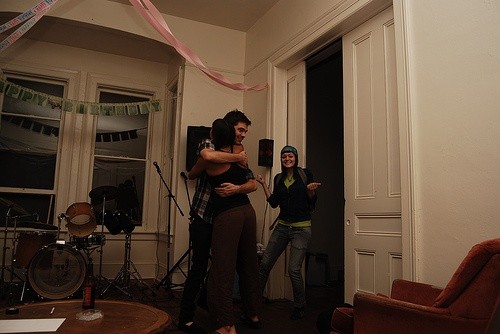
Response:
[281,145,298,156]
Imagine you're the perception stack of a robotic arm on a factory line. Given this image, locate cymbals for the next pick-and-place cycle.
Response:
[19,220,61,230]
[89,185,119,201]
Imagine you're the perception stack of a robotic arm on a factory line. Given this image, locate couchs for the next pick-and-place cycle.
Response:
[332,238,500,334]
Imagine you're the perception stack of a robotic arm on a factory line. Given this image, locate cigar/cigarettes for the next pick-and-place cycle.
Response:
[50,306,56,314]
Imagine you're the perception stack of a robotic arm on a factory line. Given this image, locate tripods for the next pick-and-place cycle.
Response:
[99,170,193,300]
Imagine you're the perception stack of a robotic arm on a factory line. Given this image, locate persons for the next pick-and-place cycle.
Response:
[243,146,321,321]
[177,108,263,334]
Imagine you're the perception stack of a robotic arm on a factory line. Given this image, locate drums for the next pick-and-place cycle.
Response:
[66,202,97,237]
[28,243,87,300]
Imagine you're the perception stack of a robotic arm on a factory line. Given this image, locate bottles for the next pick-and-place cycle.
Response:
[82,263,96,309]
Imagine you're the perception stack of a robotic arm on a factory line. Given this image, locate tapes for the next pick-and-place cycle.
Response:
[6,307,20,315]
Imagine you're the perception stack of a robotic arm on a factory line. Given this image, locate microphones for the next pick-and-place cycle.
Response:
[180,172,188,180]
[153,162,161,173]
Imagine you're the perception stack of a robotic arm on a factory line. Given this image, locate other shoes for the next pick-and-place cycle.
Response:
[197,300,208,309]
[180,321,204,334]
[242,318,263,326]
[291,308,305,319]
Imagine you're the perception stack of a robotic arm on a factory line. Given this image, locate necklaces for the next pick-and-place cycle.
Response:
[287,176,293,182]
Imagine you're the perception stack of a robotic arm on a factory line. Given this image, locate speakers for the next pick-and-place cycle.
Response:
[258,139,274,167]
[186,126,213,172]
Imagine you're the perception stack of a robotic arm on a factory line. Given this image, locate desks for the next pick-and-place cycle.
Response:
[0,300,172,334]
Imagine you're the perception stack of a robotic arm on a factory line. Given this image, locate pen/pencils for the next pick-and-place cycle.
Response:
[50,306,56,315]
